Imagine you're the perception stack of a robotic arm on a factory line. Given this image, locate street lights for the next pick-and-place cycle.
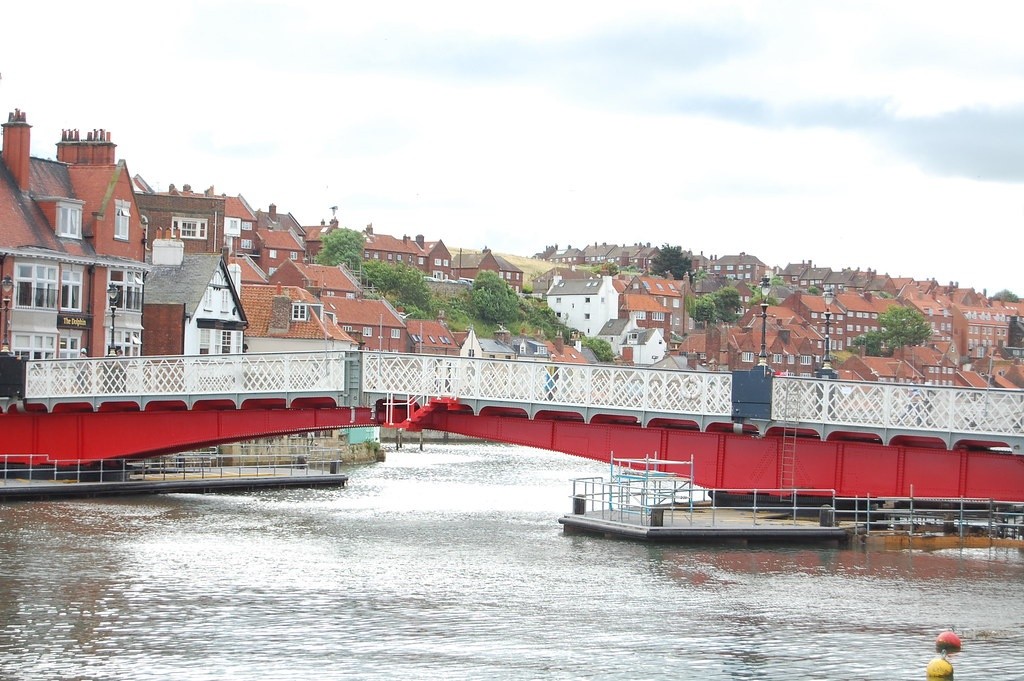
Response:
[107,282,119,357]
[818,289,839,379]
[1,273,14,356]
[757,275,772,367]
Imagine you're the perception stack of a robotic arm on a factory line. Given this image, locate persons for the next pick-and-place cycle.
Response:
[546,354,557,402]
[897,376,923,428]
[75,348,88,394]
[114,350,126,393]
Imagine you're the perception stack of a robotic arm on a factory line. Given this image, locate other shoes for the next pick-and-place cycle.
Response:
[917,424,924,427]
[897,418,900,424]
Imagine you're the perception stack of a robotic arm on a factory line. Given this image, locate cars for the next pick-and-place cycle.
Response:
[423,276,474,286]
